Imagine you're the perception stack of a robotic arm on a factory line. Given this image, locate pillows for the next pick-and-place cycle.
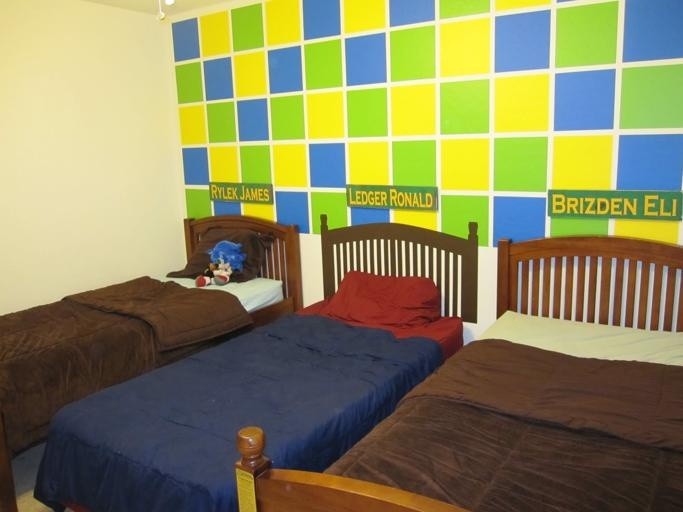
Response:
[170,224,276,285]
[324,272,442,329]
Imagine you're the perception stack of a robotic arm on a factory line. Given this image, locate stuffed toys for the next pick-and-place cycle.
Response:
[195,241,247,287]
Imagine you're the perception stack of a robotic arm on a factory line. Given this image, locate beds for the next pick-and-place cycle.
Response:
[0,214,304,512]
[234,216,682,512]
[33,216,480,512]
